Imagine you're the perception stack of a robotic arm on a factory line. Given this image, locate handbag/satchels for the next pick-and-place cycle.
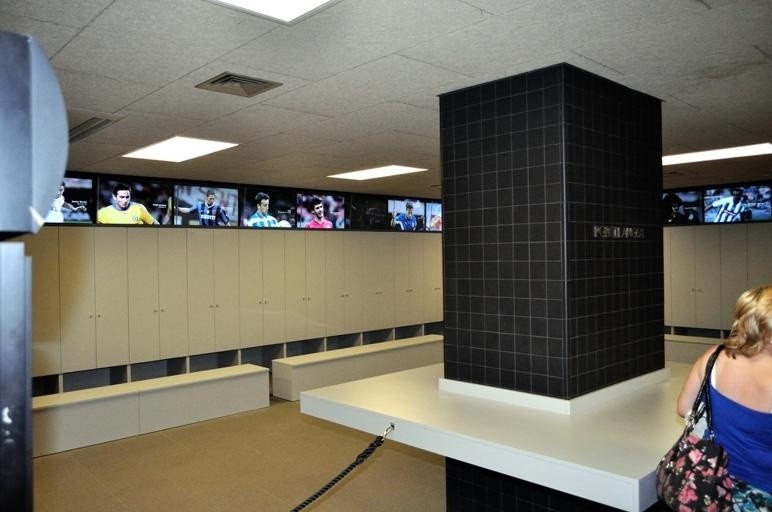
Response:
[655,433,733,511]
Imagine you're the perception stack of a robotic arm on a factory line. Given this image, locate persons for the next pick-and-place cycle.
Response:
[392,202,417,230]
[97,184,158,224]
[175,191,230,228]
[664,193,689,225]
[705,189,751,223]
[247,193,277,227]
[676,287,771,498]
[304,197,333,229]
[47,181,86,223]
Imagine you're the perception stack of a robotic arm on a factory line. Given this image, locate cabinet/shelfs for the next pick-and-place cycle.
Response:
[23,226,450,379]
[661,219,772,329]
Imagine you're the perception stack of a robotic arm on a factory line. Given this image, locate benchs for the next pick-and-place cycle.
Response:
[31,362,273,462]
[272,335,445,407]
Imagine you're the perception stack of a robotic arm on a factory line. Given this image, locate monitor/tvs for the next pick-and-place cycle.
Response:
[43,174,98,226]
[352,195,388,231]
[299,191,348,231]
[662,180,772,227]
[101,176,174,227]
[175,184,240,227]
[427,202,442,232]
[389,198,426,232]
[243,185,298,229]
